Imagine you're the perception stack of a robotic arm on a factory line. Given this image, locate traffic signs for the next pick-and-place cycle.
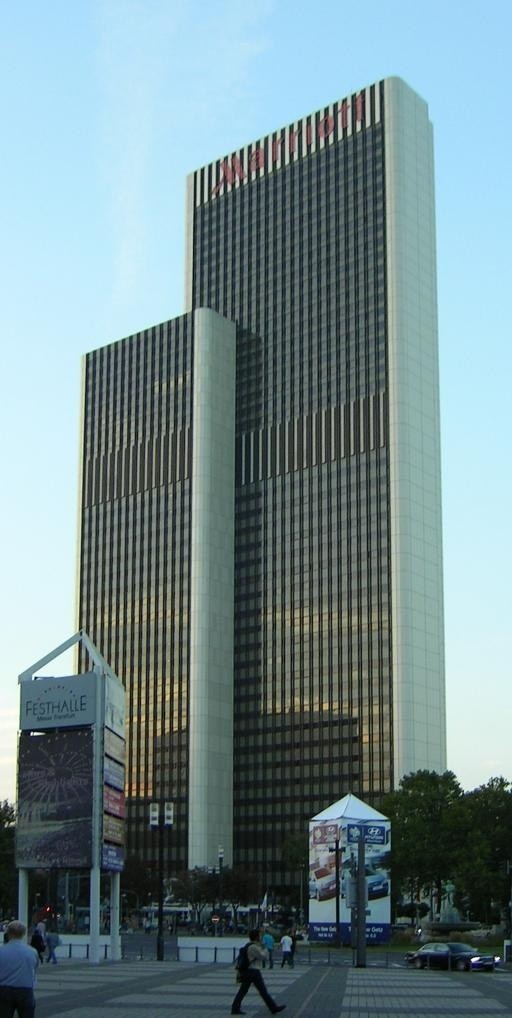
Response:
[211,915,220,924]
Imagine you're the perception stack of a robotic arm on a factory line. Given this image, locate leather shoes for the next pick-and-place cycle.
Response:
[231,1008,246,1014]
[271,1003,287,1014]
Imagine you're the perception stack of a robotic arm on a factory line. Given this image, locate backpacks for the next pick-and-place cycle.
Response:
[236,943,257,972]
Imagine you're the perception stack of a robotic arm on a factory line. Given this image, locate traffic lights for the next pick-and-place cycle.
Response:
[42,904,56,913]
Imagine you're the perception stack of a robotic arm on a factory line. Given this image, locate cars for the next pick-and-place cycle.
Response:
[404,941,502,973]
[309,867,341,901]
[340,864,388,899]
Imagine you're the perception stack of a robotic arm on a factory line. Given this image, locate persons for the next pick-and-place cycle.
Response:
[262,918,309,941]
[278,931,295,969]
[1,920,41,1018]
[1,920,10,943]
[186,920,249,936]
[121,917,129,935]
[30,928,45,965]
[28,910,89,934]
[260,929,274,967]
[230,929,287,1015]
[143,916,151,936]
[45,928,62,964]
[289,931,296,964]
